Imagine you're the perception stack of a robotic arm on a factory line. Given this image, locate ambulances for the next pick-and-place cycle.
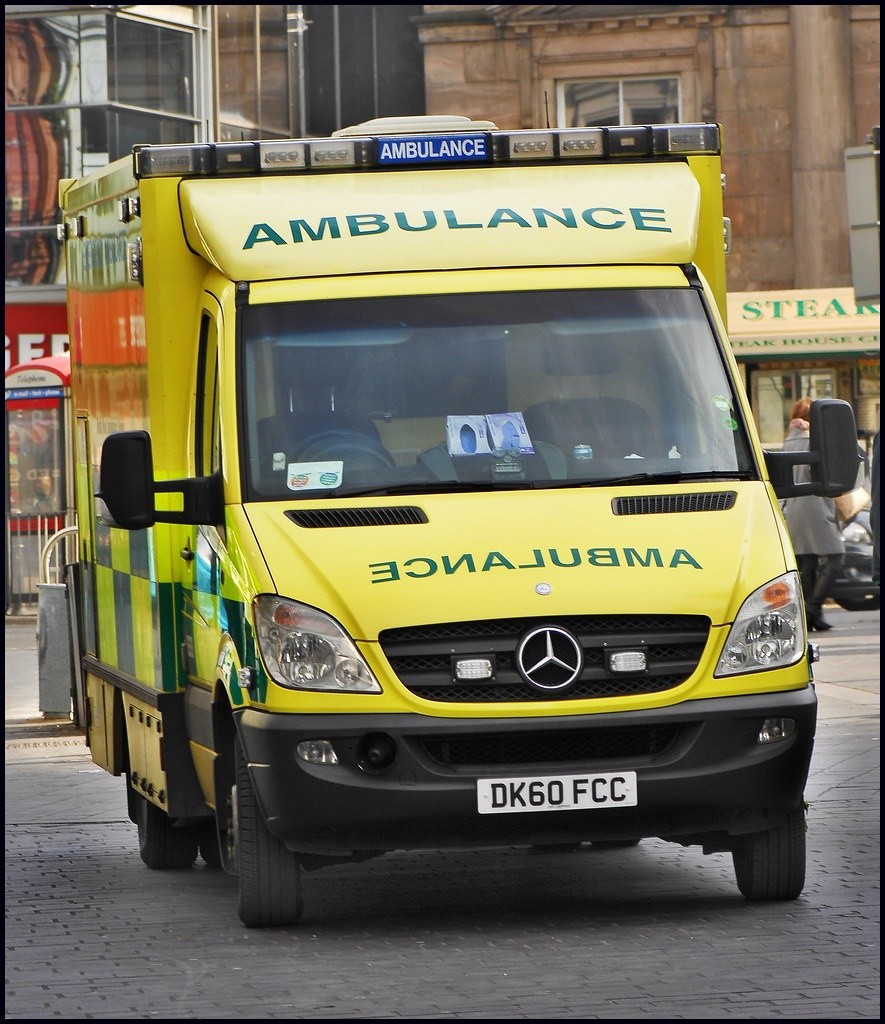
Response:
[58,115,859,929]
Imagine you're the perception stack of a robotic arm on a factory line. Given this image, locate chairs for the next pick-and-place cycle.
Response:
[515,399,656,461]
[256,417,393,486]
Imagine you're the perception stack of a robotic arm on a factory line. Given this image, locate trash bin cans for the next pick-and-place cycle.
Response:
[36,583,74,719]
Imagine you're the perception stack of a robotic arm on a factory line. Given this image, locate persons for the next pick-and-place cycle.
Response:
[780,396,851,630]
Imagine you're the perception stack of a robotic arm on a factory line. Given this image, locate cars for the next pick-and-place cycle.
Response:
[829,507,881,613]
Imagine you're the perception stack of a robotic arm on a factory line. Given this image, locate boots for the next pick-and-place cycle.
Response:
[807,601,834,631]
